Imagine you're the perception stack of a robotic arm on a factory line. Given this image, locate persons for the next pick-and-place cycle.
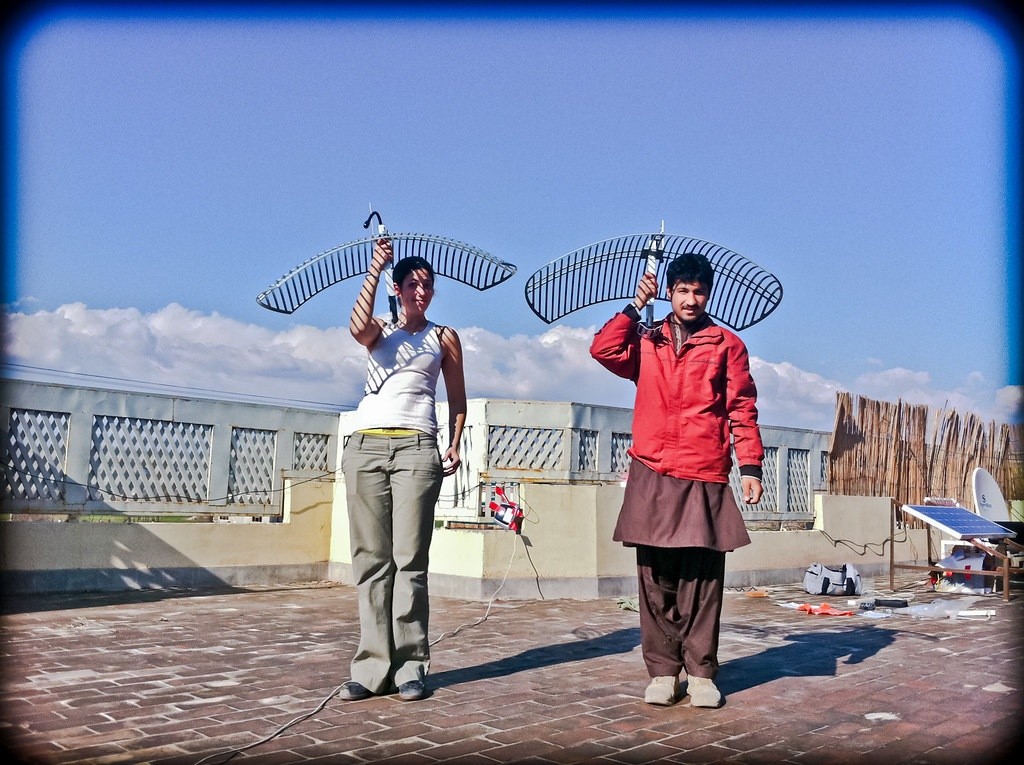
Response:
[340,238,468,700]
[589,252,765,707]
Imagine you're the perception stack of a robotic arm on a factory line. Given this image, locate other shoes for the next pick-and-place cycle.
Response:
[339,681,370,700]
[398,680,424,700]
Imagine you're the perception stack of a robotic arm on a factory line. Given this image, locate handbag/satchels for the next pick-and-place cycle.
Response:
[803,563,863,597]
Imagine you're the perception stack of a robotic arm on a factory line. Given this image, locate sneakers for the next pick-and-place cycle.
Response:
[645,677,679,706]
[687,673,722,708]
